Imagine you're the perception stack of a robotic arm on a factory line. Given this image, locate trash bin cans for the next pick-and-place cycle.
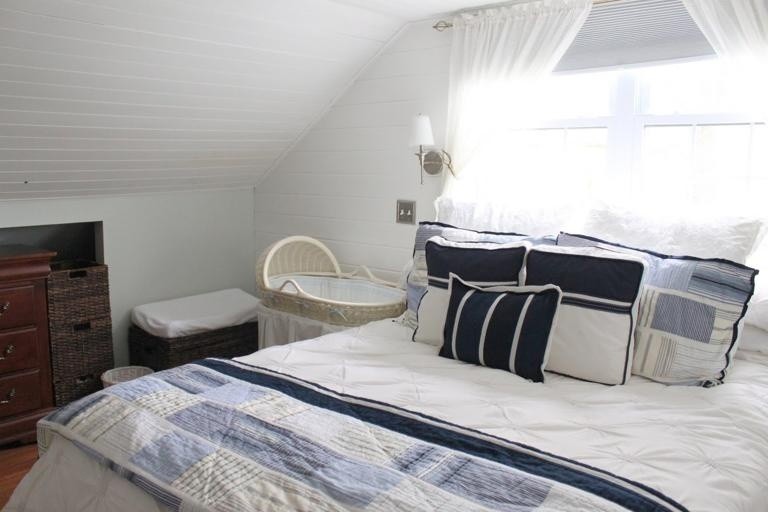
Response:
[101,366,154,388]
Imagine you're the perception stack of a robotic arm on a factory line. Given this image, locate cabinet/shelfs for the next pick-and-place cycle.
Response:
[43,258,114,408]
[0,245,57,447]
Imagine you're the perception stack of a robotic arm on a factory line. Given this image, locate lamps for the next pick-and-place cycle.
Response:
[411,111,454,186]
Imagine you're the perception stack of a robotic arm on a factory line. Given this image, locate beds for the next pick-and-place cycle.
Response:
[0,199,768,512]
[254,233,408,350]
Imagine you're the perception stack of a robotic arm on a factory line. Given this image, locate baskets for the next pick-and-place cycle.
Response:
[47,264,115,406]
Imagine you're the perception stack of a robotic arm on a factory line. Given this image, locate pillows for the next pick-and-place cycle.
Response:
[518,243,648,388]
[410,233,533,351]
[582,208,764,269]
[390,220,530,332]
[437,269,562,387]
[430,195,554,236]
[550,227,758,389]
